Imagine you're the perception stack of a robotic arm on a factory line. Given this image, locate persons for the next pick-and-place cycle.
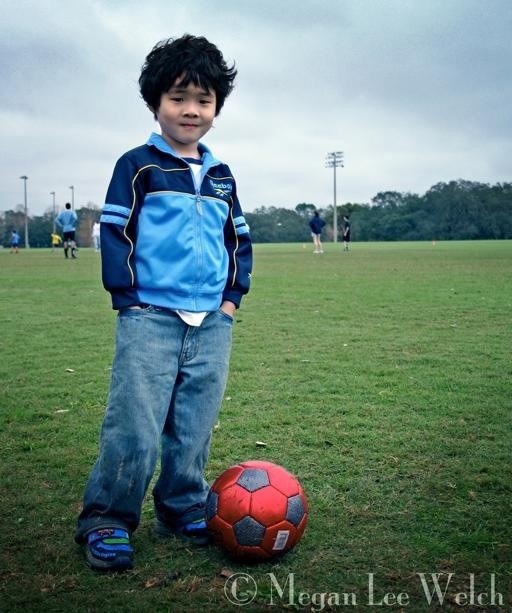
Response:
[339,214,352,250]
[9,228,21,255]
[308,209,326,255]
[49,231,63,255]
[89,219,101,252]
[55,202,78,258]
[72,29,253,573]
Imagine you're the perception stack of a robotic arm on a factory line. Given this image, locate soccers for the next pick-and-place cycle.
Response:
[204,461,308,563]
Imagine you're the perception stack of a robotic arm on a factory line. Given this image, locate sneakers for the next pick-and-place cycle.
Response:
[81,528,134,570]
[154,517,209,546]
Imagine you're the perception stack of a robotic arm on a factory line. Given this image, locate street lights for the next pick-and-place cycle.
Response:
[323,147,345,244]
[20,174,32,248]
[50,189,56,248]
[68,184,75,212]
